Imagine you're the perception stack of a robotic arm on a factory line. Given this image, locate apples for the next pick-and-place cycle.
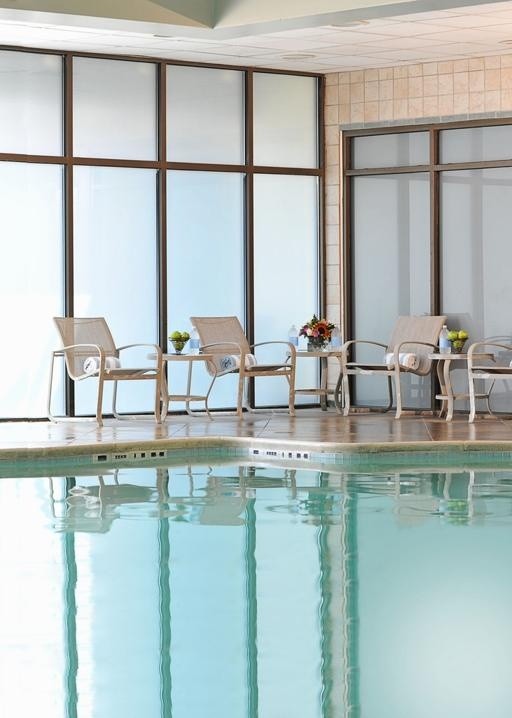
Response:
[447,330,468,349]
[171,331,189,351]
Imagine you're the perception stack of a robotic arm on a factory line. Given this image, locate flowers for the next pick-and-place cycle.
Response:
[298,314,336,348]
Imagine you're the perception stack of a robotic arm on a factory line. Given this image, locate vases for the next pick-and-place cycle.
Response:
[307,339,328,352]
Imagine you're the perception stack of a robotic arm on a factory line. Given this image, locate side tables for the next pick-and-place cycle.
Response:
[428,352,500,420]
[147,352,218,421]
[284,349,350,414]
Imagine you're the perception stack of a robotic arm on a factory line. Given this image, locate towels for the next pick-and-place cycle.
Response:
[82,356,122,373]
[385,352,421,371]
[220,353,258,370]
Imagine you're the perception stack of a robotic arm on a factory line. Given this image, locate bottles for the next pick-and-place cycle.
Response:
[288,325,298,352]
[189,327,199,355]
[439,325,451,354]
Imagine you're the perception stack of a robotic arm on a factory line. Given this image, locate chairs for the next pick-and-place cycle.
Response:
[466,336,512,423]
[187,316,296,421]
[48,317,163,428]
[49,464,512,533]
[342,314,448,419]
